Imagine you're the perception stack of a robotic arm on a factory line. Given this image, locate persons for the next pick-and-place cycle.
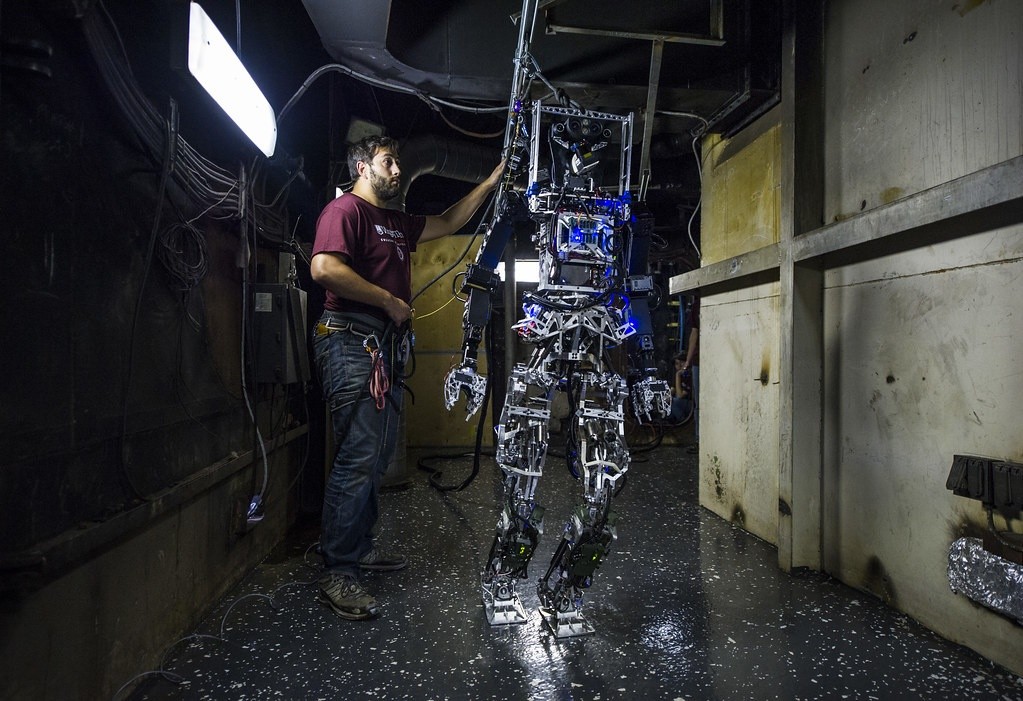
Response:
[311,135,508,619]
[657,304,700,454]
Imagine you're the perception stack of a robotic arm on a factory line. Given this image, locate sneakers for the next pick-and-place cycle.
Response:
[317,570,381,620]
[356,542,408,570]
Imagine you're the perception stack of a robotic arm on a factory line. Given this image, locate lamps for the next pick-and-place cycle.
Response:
[182,0,281,163]
